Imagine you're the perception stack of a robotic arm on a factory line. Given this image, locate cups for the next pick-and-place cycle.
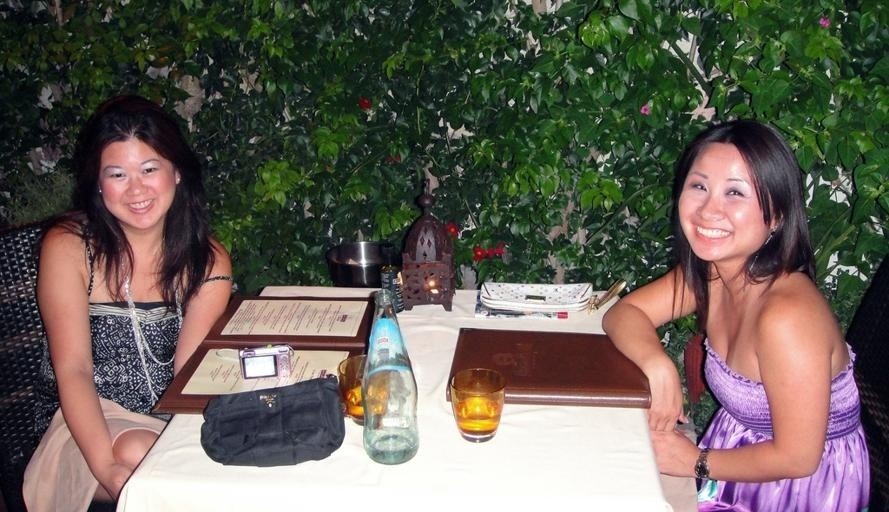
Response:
[451,368,506,443]
[337,355,391,430]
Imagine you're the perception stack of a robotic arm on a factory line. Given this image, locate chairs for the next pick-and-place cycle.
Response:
[0,213,64,489]
[842,249,889,512]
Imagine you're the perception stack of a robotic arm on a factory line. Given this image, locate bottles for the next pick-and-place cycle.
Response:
[361,290,417,465]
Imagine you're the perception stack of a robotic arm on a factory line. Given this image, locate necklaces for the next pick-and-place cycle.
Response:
[107,241,184,366]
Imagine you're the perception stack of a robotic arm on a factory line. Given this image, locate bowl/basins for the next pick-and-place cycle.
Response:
[326,241,394,287]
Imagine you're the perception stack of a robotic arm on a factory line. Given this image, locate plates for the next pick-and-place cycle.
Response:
[481,281,592,312]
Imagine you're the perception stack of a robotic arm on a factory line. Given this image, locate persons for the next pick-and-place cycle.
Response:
[600,118,871,512]
[21,92,225,511]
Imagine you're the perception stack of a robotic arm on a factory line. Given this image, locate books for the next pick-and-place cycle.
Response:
[445,324,654,411]
[150,290,377,417]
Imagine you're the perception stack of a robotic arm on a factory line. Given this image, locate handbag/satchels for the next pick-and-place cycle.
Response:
[200,373,345,467]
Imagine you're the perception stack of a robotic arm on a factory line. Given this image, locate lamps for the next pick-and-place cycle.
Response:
[398,182,457,312]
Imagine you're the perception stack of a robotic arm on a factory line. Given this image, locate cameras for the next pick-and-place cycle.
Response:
[237,344,292,382]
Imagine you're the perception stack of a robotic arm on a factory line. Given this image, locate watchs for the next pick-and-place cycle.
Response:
[692,446,712,483]
